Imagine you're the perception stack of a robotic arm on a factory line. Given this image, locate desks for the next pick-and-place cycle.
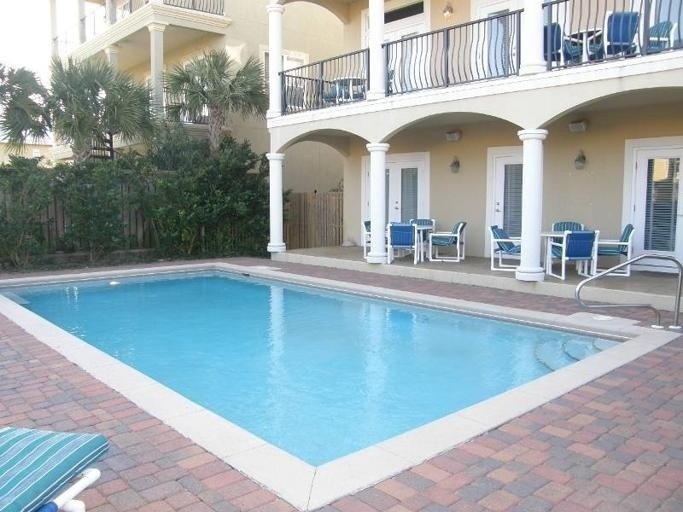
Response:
[334,78,364,103]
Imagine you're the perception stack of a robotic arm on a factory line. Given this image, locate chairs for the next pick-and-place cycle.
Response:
[0,423,110,512]
[490,222,634,281]
[364,218,466,265]
[316,80,347,103]
[544,13,678,68]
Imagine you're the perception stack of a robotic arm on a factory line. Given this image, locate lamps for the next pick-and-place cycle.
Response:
[446,130,460,141]
[443,6,453,16]
[569,120,588,132]
[574,150,585,169]
[450,156,459,173]
